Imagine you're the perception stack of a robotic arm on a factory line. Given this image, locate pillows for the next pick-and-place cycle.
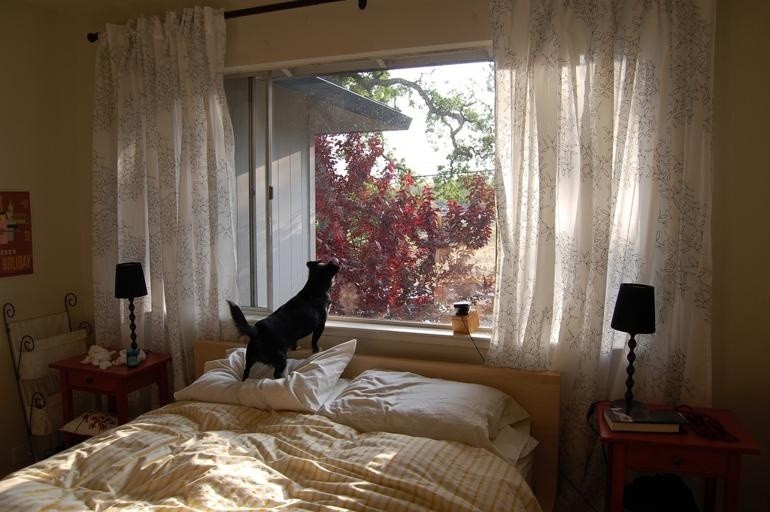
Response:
[330,369,540,468]
[173,339,358,414]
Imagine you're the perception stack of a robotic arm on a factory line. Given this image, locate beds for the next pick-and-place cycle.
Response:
[0,340,563,512]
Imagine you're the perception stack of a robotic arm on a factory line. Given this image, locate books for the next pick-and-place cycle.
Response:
[603,406,689,435]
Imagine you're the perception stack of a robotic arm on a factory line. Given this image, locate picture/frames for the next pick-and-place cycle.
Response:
[1,185,40,281]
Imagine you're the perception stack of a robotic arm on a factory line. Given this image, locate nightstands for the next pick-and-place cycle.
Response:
[594,399,758,511]
[47,348,173,449]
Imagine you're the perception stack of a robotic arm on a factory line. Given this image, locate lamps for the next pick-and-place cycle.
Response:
[610,282,657,409]
[113,261,149,352]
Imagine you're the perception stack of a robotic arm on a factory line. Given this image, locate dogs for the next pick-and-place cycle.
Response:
[226,260,341,382]
